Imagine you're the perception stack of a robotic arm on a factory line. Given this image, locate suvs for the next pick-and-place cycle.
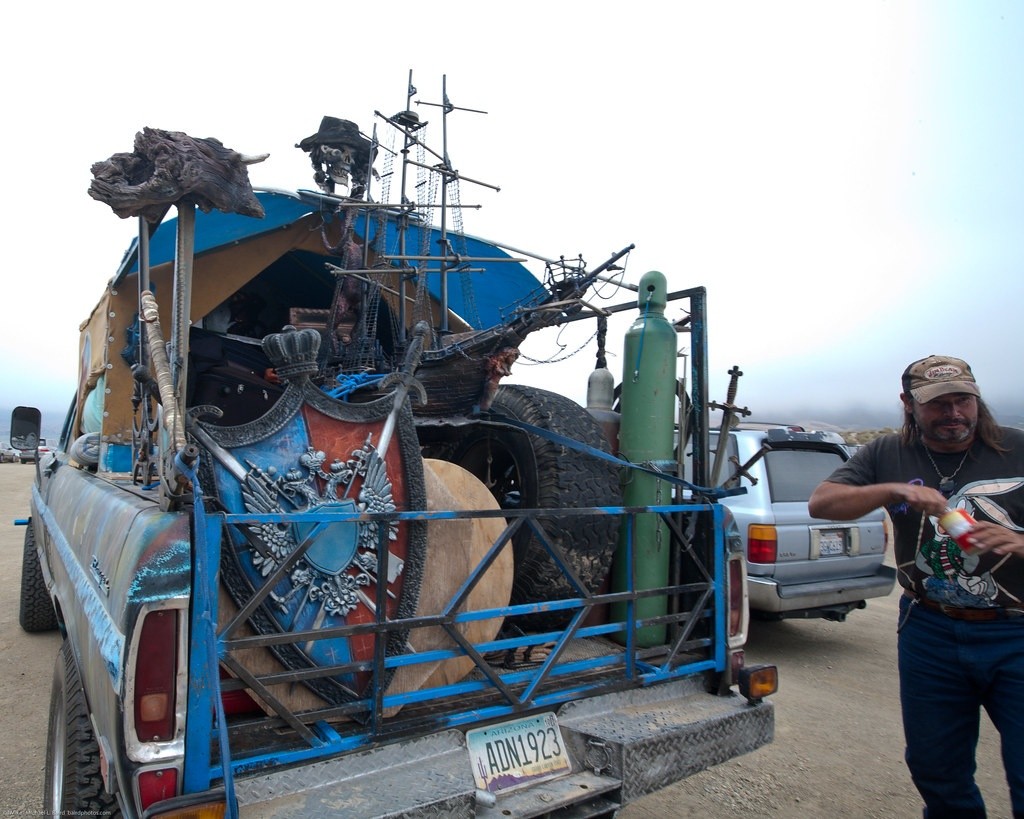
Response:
[671,419,897,635]
[20,441,57,464]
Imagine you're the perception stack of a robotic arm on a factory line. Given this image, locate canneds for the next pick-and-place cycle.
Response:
[937,508,992,557]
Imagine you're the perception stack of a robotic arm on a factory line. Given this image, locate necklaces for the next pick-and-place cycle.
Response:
[921,440,970,493]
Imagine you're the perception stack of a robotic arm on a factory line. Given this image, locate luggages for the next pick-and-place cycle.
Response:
[191,366,284,427]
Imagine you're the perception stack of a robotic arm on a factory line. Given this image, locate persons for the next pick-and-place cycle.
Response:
[808,354,1024,819]
[300,116,377,187]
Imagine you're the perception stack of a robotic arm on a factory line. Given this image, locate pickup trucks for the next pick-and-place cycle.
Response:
[9,179,778,819]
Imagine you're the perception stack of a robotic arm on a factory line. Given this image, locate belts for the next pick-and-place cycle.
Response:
[903,588,1024,621]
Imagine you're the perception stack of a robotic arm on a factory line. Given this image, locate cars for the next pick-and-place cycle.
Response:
[0,441,17,463]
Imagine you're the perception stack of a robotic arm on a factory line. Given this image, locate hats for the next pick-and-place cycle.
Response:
[901,355,981,405]
